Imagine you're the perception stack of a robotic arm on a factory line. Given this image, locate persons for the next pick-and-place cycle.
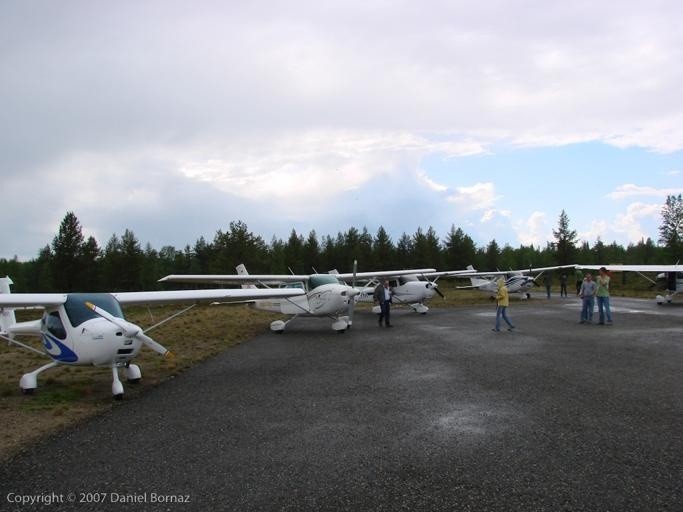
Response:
[558,272,567,297]
[579,274,597,324]
[574,269,584,296]
[491,278,515,332]
[542,272,552,298]
[372,280,396,326]
[595,267,612,325]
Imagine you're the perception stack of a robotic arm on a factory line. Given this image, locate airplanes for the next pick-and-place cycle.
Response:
[575,265,683,305]
[158,264,577,333]
[0,275,306,400]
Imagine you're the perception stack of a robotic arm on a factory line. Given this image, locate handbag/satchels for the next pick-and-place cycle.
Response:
[372,306,381,313]
